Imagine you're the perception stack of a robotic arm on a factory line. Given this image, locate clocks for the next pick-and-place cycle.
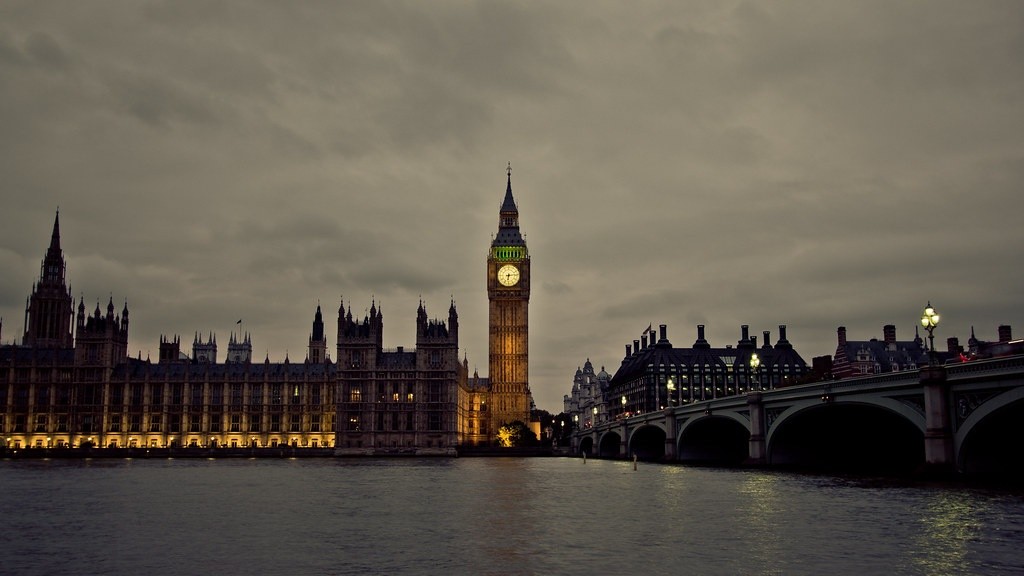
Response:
[498,264,520,287]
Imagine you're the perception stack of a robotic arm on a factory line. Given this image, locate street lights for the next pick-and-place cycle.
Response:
[622,395,627,416]
[594,407,598,426]
[667,379,674,407]
[574,415,578,432]
[920,301,941,365]
[749,352,760,390]
